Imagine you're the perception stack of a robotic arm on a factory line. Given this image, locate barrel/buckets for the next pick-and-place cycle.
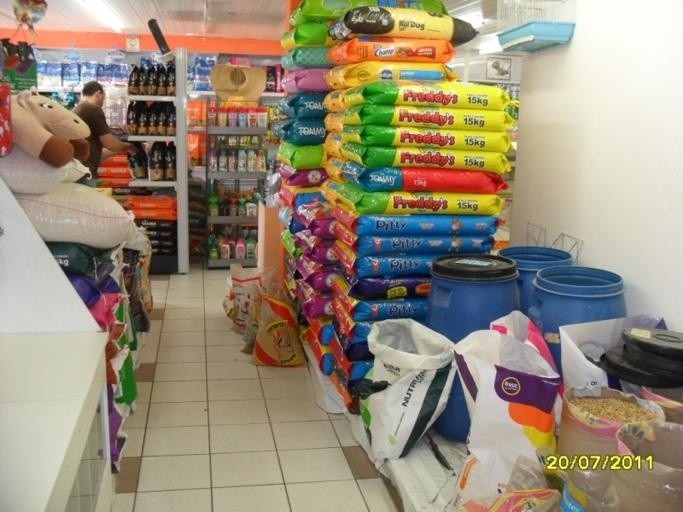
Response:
[426,253,521,443]
[500,246,572,316]
[530,266,627,373]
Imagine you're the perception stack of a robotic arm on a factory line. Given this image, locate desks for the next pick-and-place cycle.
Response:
[0,330,115,511]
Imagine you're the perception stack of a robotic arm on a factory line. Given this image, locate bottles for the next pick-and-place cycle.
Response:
[207,222,257,261]
[206,106,267,126]
[206,148,266,173]
[207,190,261,218]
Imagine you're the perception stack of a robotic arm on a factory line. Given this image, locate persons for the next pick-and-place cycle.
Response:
[70,82,138,187]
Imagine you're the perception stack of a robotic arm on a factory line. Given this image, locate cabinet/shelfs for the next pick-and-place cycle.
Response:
[128,48,185,274]
[185,85,290,261]
[35,77,127,133]
[205,122,265,269]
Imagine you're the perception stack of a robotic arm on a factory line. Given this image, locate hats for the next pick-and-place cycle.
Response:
[210,63,267,102]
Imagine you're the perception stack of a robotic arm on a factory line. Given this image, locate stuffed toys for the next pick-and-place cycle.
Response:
[7,86,90,169]
